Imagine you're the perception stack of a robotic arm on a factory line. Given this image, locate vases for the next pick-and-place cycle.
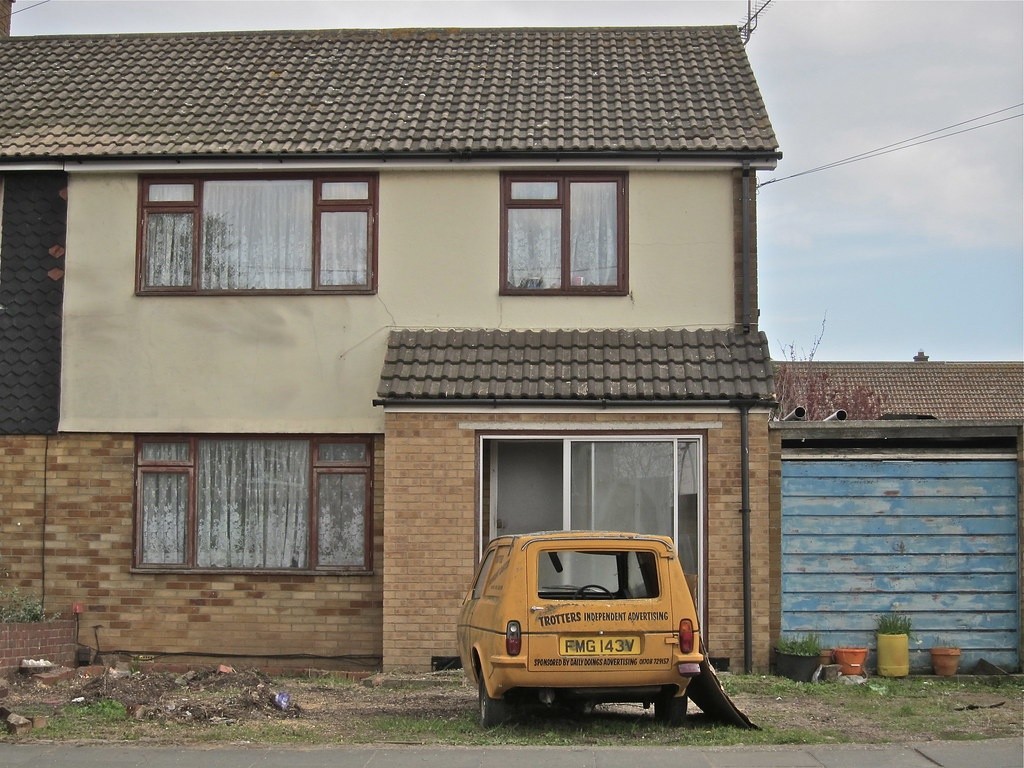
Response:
[837,646,867,675]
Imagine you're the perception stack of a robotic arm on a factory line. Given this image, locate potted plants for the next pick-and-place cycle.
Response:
[872,611,915,677]
[820,645,833,664]
[773,630,820,684]
[930,637,961,676]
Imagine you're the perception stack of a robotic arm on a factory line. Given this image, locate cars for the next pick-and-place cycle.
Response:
[455,528,704,729]
